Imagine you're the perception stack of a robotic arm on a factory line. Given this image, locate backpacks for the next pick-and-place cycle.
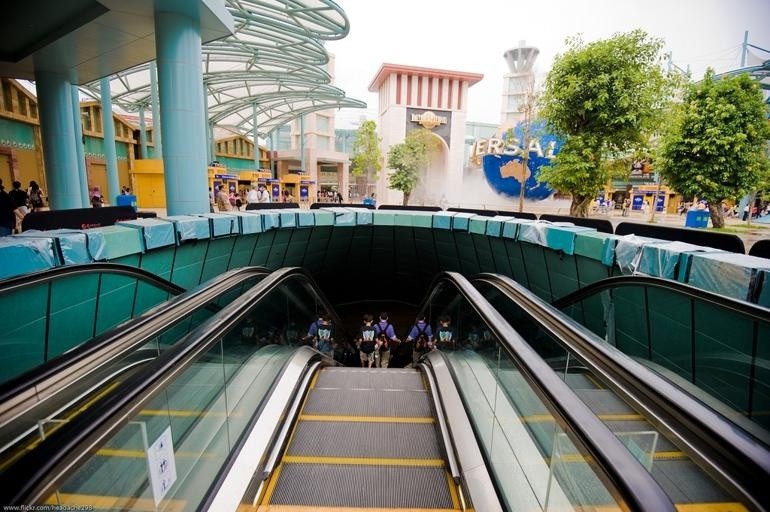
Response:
[375,323,392,353]
[414,324,430,352]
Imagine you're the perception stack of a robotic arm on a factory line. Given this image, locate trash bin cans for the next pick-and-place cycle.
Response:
[117,195,137,214]
[364,199,373,205]
[686,209,709,227]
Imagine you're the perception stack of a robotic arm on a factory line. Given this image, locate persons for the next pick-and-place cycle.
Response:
[375,311,401,367]
[92,186,103,203]
[356,314,383,367]
[121,184,126,194]
[403,314,434,363]
[312,315,338,358]
[229,185,295,211]
[27,180,44,212]
[209,187,216,212]
[124,188,131,195]
[216,185,232,211]
[336,193,343,203]
[30,183,43,212]
[434,316,457,350]
[317,191,321,201]
[9,181,31,234]
[299,311,323,348]
[91,195,104,208]
[592,197,770,221]
[1,179,24,239]
